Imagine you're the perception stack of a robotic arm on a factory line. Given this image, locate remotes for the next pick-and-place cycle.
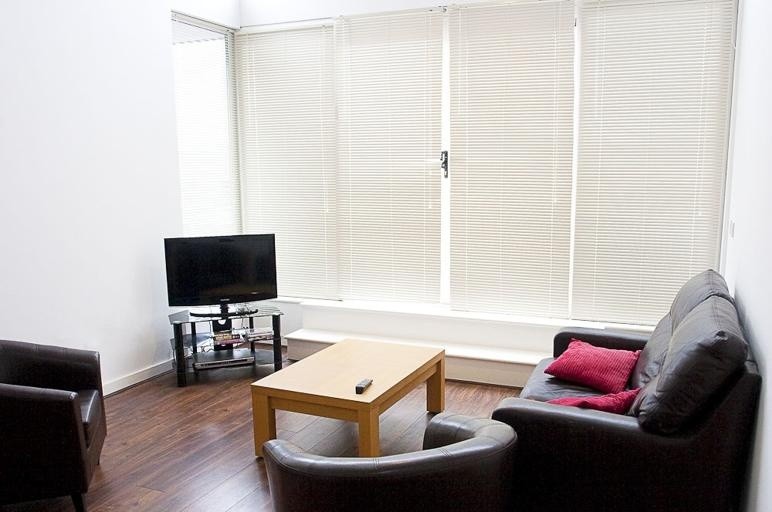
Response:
[355,379,373,394]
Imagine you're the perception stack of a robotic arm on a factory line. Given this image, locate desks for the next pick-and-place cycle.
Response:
[168,306,284,387]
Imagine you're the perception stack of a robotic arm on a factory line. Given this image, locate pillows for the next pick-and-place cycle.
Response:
[542,338,642,416]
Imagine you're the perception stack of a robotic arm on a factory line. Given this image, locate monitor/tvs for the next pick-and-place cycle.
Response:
[164,233,278,317]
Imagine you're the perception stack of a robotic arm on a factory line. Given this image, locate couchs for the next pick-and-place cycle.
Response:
[492,269,761,511]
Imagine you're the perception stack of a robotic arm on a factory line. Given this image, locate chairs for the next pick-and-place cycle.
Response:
[0,338,108,512]
[262,413,519,510]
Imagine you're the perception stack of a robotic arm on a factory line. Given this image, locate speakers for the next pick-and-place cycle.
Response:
[212,319,233,350]
